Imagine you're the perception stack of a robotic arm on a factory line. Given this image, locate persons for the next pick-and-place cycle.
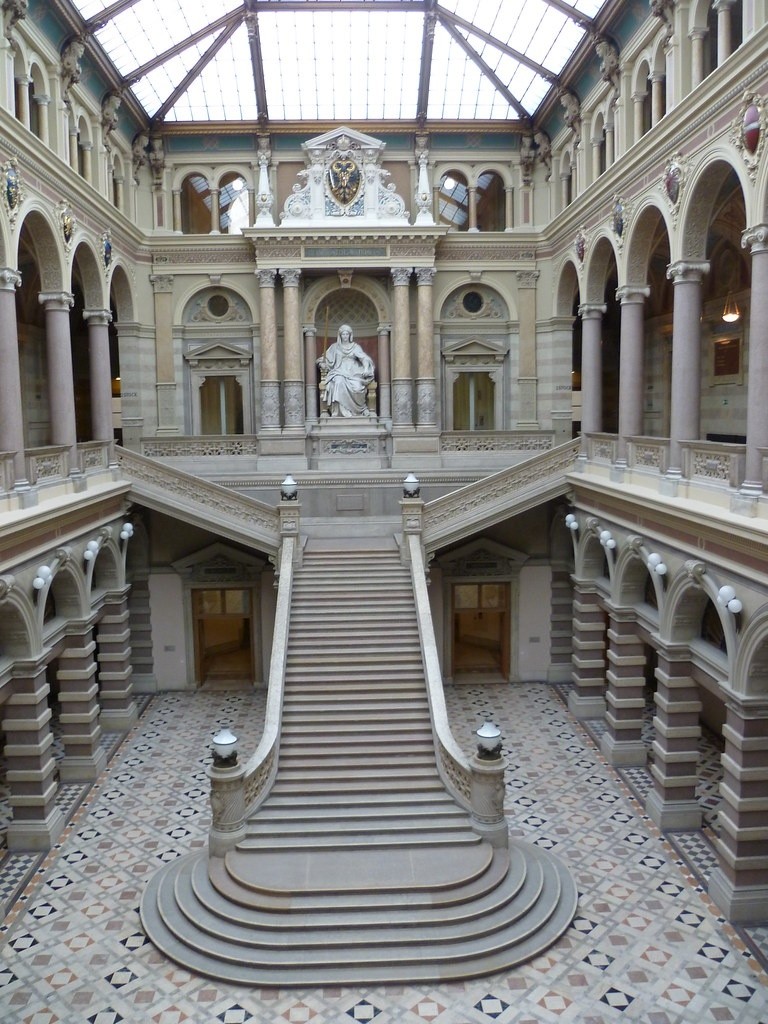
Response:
[520,0,670,164]
[0,0,164,171]
[316,325,375,416]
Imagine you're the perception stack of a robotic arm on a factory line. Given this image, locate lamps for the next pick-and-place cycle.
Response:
[83,540,99,575]
[281,474,298,498]
[212,729,237,759]
[599,530,617,564]
[721,198,740,322]
[476,717,501,751]
[120,522,134,554]
[33,565,52,607]
[565,514,579,544]
[716,586,743,636]
[403,472,419,495]
[646,553,668,592]
